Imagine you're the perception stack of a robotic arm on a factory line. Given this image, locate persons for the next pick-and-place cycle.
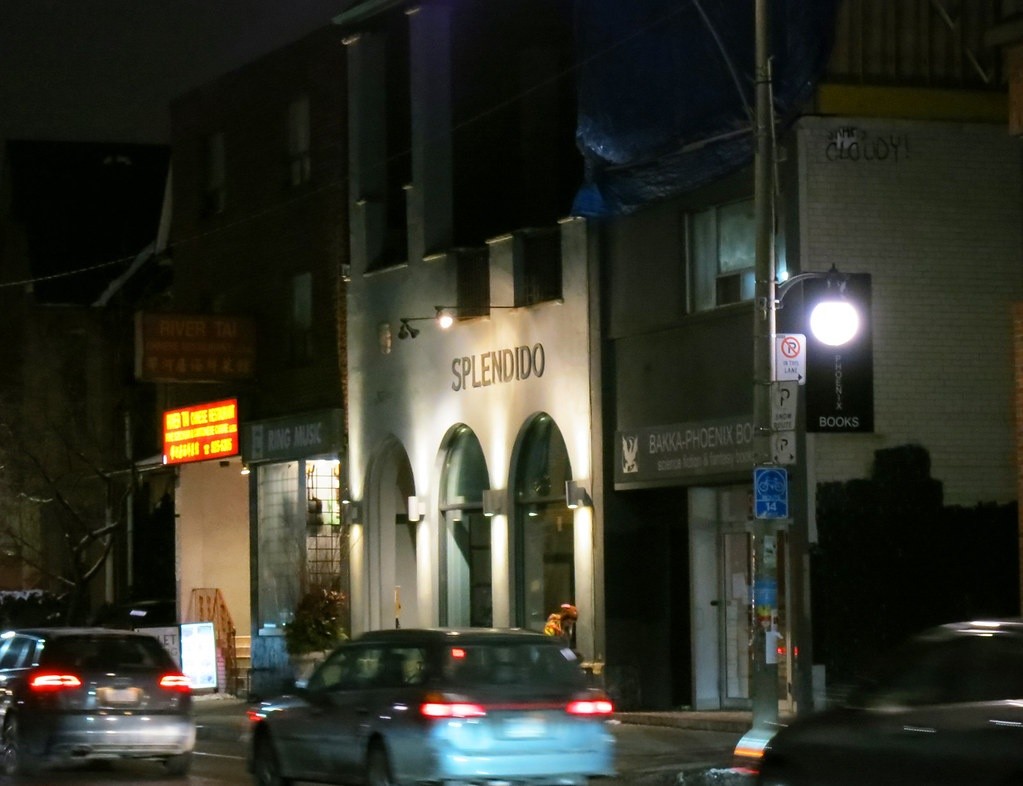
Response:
[544,604,578,643]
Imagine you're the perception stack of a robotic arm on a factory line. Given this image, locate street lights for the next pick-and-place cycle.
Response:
[776,268,864,722]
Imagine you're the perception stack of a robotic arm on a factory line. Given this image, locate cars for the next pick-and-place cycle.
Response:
[242,626,621,786]
[751,694,1022,786]
[1,625,199,782]
[885,615,1023,716]
